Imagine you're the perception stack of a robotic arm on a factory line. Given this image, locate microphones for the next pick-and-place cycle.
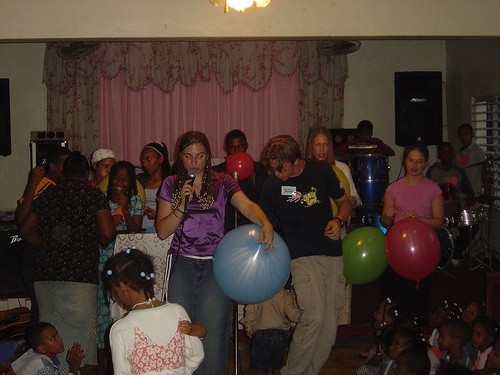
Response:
[184,170,197,212]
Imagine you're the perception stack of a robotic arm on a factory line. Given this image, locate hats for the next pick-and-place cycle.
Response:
[92,148,116,163]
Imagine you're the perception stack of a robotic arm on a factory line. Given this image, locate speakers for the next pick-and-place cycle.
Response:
[30,140,68,170]
[0,218,37,298]
[394,71,444,146]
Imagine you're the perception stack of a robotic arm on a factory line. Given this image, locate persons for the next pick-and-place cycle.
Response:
[357,296,500,375]
[237,287,302,375]
[209,128,270,232]
[259,134,354,375]
[383,144,444,230]
[455,123,487,195]
[0,147,208,375]
[304,125,362,326]
[135,139,174,232]
[336,119,396,156]
[426,142,474,256]
[157,132,274,375]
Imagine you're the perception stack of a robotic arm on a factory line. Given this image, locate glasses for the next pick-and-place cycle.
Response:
[184,154,205,160]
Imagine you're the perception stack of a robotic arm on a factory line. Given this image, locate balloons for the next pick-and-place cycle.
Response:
[226,149,255,181]
[341,227,388,287]
[384,217,441,289]
[212,223,290,304]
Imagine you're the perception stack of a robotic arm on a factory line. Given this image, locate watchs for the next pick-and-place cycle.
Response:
[336,215,346,230]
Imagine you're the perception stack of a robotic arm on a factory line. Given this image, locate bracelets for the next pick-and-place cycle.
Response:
[172,207,187,220]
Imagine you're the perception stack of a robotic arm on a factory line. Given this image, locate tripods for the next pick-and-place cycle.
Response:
[456,161,500,274]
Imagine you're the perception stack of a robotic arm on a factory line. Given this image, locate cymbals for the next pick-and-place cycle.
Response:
[464,155,500,168]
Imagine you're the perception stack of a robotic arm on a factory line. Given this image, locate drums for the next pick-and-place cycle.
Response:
[437,217,462,273]
[336,152,389,213]
[455,208,486,229]
